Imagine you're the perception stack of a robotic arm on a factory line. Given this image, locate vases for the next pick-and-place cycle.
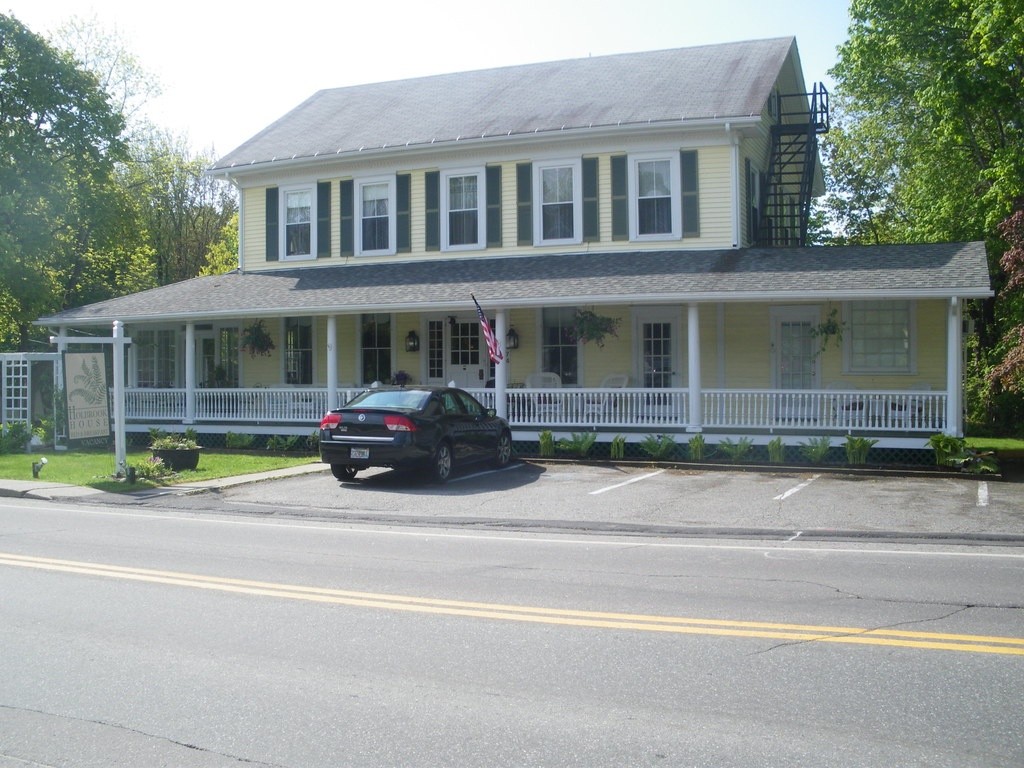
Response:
[397,379,406,385]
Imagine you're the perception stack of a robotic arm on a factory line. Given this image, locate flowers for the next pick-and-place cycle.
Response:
[395,370,412,385]
[566,311,620,347]
[239,318,276,360]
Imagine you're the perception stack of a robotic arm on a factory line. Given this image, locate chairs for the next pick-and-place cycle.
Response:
[585,375,628,417]
[827,381,931,428]
[526,372,563,416]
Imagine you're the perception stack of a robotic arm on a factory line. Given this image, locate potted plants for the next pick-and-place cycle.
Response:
[148,427,203,473]
[809,309,848,358]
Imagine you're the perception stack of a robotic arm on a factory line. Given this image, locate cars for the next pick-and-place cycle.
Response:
[316,382,514,486]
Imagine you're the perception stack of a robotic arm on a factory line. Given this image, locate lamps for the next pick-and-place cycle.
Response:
[505,329,518,349]
[32,457,47,478]
[405,331,419,352]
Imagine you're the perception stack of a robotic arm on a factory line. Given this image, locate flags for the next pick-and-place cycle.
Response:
[475,301,504,366]
